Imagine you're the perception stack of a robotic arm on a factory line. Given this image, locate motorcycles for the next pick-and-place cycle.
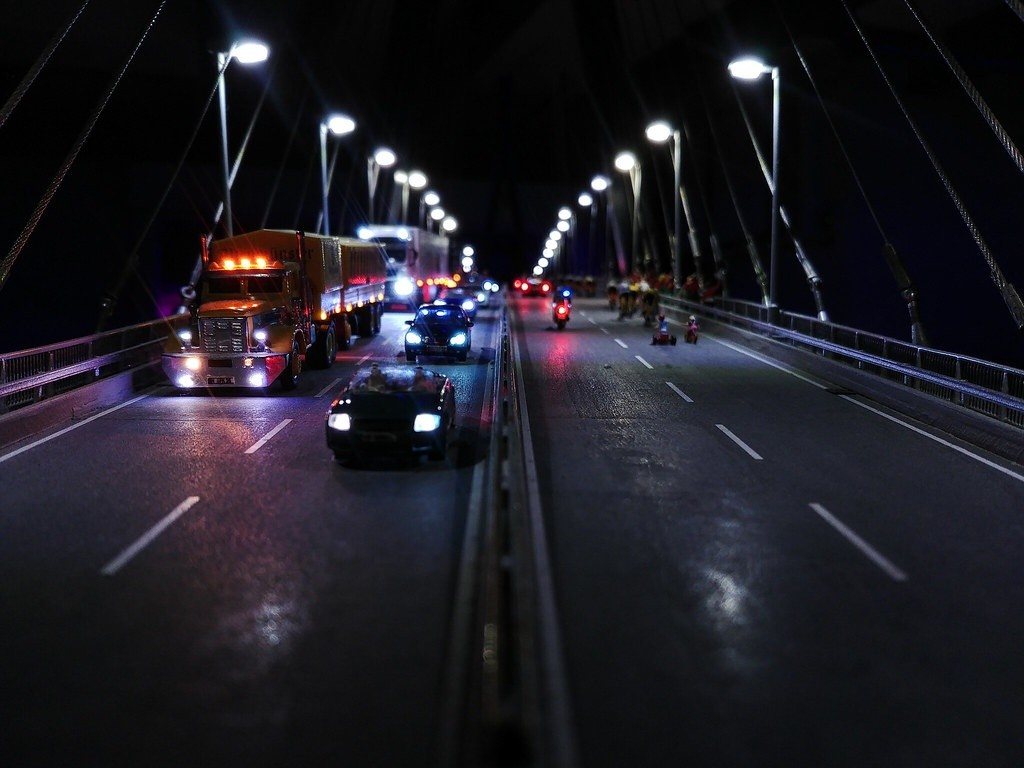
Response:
[553,291,573,331]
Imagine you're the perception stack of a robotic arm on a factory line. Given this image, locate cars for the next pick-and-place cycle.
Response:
[520,276,550,298]
[432,288,479,327]
[463,275,499,309]
[404,303,475,362]
[325,365,456,453]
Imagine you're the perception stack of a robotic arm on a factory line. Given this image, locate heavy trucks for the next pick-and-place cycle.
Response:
[359,223,449,313]
[160,227,387,395]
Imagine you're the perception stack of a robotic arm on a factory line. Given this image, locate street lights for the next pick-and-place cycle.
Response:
[424,206,445,232]
[418,189,439,228]
[646,120,681,299]
[616,154,642,273]
[217,42,271,240]
[729,60,786,338]
[594,177,614,280]
[366,147,397,226]
[401,170,427,224]
[320,116,360,237]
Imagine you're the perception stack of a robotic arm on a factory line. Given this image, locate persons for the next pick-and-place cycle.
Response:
[607,272,720,321]
[682,316,701,339]
[654,314,672,340]
[553,285,572,322]
